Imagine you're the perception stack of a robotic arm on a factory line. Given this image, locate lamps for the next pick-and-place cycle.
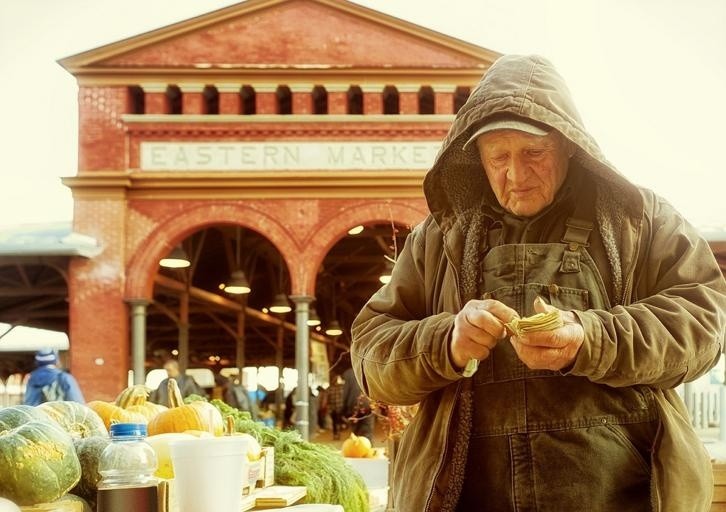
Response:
[158,226,344,337]
[378,199,400,285]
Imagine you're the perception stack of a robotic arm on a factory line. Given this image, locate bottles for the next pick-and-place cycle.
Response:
[95,423,158,491]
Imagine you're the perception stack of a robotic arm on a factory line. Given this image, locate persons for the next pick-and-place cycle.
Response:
[350,51,725,510]
[143,356,373,440]
[20,349,86,408]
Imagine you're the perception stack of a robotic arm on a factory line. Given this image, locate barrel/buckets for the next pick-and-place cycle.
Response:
[167,434,252,512]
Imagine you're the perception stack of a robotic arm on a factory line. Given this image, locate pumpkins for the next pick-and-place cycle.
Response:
[0,378,261,512]
[342,432,372,458]
[364,448,380,458]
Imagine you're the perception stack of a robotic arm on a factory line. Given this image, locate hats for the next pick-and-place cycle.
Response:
[460,119,552,154]
[34,347,56,362]
